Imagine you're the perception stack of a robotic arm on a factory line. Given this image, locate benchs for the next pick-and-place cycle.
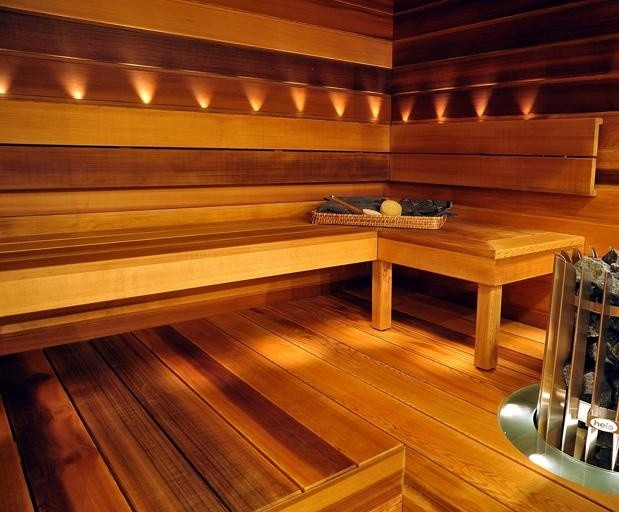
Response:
[0,216,585,371]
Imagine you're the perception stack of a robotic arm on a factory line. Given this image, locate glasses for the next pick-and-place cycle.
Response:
[399,199,438,216]
